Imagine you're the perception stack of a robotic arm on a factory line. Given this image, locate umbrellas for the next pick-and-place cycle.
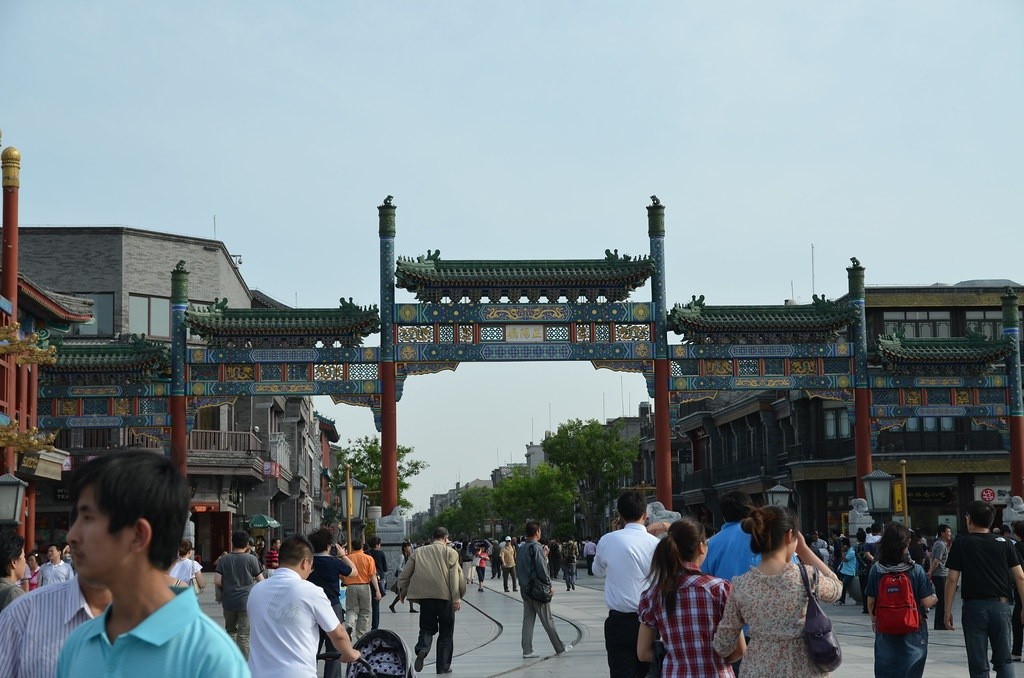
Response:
[466,539,493,559]
[243,513,281,528]
[646,521,671,537]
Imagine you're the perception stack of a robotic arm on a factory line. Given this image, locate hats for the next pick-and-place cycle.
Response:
[646,501,681,532]
[491,540,498,544]
[505,536,511,541]
[993,528,1000,533]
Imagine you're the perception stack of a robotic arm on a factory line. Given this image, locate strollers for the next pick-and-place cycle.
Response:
[313,626,416,678]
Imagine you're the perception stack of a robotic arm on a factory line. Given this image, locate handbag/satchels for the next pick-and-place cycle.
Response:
[525,577,552,603]
[471,556,482,566]
[796,563,842,672]
[191,559,204,595]
[370,573,387,600]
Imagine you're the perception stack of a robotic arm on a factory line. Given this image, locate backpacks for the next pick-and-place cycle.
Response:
[875,572,925,634]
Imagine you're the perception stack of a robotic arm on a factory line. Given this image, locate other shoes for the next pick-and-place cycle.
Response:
[491,575,495,579]
[513,590,518,591]
[478,589,484,592]
[410,609,419,613]
[505,590,510,592]
[1012,658,1021,662]
[567,588,570,591]
[415,650,425,672]
[497,575,500,578]
[389,604,396,613]
[523,652,540,658]
[572,583,575,590]
[446,669,452,672]
[558,645,573,656]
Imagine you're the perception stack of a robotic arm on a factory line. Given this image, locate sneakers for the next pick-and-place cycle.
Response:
[833,601,845,606]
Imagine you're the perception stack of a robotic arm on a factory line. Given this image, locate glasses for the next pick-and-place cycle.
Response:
[506,540,511,542]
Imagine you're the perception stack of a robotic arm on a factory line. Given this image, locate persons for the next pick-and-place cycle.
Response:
[592,491,661,678]
[396,526,466,675]
[698,491,799,678]
[169,531,595,661]
[0,529,77,614]
[944,501,1024,678]
[806,520,1024,662]
[637,517,746,678]
[516,521,573,659]
[57,449,252,678]
[247,533,361,678]
[306,528,358,678]
[0,508,189,678]
[864,521,938,678]
[712,505,843,678]
